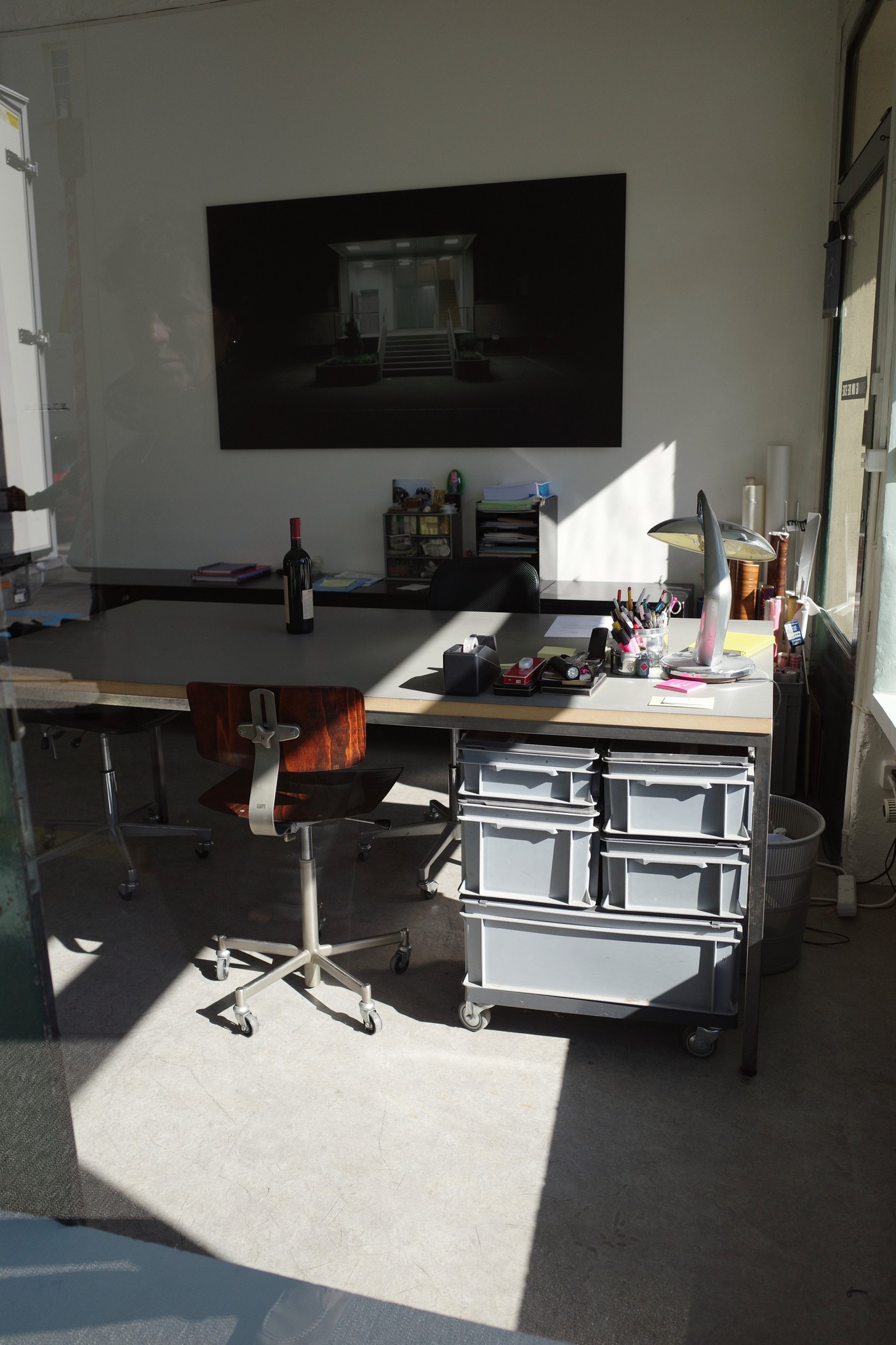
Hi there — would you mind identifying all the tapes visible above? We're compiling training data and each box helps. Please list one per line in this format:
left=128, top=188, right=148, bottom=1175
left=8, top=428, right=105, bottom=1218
left=463, top=637, right=479, bottom=652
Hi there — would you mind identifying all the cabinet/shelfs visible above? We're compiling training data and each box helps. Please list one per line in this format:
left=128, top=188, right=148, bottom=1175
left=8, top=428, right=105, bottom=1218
left=382, top=509, right=461, bottom=580
left=478, top=497, right=558, bottom=579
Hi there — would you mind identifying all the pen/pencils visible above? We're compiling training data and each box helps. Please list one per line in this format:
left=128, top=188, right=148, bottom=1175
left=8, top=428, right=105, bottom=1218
left=611, top=587, right=678, bottom=629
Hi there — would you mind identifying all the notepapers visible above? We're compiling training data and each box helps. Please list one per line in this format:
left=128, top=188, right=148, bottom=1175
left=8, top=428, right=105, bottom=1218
left=657, top=678, right=707, bottom=693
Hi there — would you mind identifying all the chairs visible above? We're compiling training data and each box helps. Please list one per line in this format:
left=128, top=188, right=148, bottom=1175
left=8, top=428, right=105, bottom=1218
left=428, top=558, right=542, bottom=613
left=186, top=680, right=411, bottom=1035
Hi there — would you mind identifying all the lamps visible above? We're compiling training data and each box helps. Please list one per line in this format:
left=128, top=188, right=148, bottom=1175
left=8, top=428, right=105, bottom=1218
left=651, top=491, right=778, bottom=680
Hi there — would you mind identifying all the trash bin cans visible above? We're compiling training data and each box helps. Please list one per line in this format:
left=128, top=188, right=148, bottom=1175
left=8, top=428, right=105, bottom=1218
left=762, top=795, right=826, bottom=971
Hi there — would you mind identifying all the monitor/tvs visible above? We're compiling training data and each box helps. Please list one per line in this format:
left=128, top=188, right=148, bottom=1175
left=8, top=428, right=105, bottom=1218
left=206, top=171, right=627, bottom=450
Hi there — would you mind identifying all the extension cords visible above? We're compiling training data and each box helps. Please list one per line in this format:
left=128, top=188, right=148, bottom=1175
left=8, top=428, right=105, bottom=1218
left=836, top=874, right=857, bottom=917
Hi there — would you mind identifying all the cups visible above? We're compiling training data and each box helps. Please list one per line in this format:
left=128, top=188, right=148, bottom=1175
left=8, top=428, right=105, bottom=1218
left=610, top=646, right=647, bottom=676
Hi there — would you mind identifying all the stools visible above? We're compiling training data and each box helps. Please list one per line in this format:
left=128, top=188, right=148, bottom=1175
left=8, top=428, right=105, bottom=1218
left=39, top=704, right=220, bottom=901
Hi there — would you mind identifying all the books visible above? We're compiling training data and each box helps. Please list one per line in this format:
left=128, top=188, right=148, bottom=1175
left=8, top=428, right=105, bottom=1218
left=192, top=562, right=271, bottom=582
left=477, top=499, right=538, bottom=558
left=656, top=678, right=706, bottom=693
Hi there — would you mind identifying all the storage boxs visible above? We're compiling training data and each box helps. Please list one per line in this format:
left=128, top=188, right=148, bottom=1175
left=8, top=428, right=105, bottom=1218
left=452, top=739, right=755, bottom=1019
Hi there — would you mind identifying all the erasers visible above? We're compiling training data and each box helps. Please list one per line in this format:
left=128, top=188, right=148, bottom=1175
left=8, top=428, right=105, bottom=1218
left=519, top=658, right=533, bottom=669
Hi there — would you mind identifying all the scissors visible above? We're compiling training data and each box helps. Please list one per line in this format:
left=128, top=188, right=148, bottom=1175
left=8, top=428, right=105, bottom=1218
left=660, top=591, right=682, bottom=614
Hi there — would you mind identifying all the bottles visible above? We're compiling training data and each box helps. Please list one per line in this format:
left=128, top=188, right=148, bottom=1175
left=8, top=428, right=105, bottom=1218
left=283, top=517, right=314, bottom=636
left=391, top=515, right=399, bottom=535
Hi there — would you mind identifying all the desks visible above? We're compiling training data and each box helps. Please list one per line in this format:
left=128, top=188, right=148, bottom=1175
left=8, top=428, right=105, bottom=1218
left=76, top=566, right=695, bottom=620
left=0, top=600, right=770, bottom=1087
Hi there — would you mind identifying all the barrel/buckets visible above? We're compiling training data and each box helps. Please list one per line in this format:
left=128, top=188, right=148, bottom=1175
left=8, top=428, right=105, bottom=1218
left=631, top=626, right=669, bottom=668
